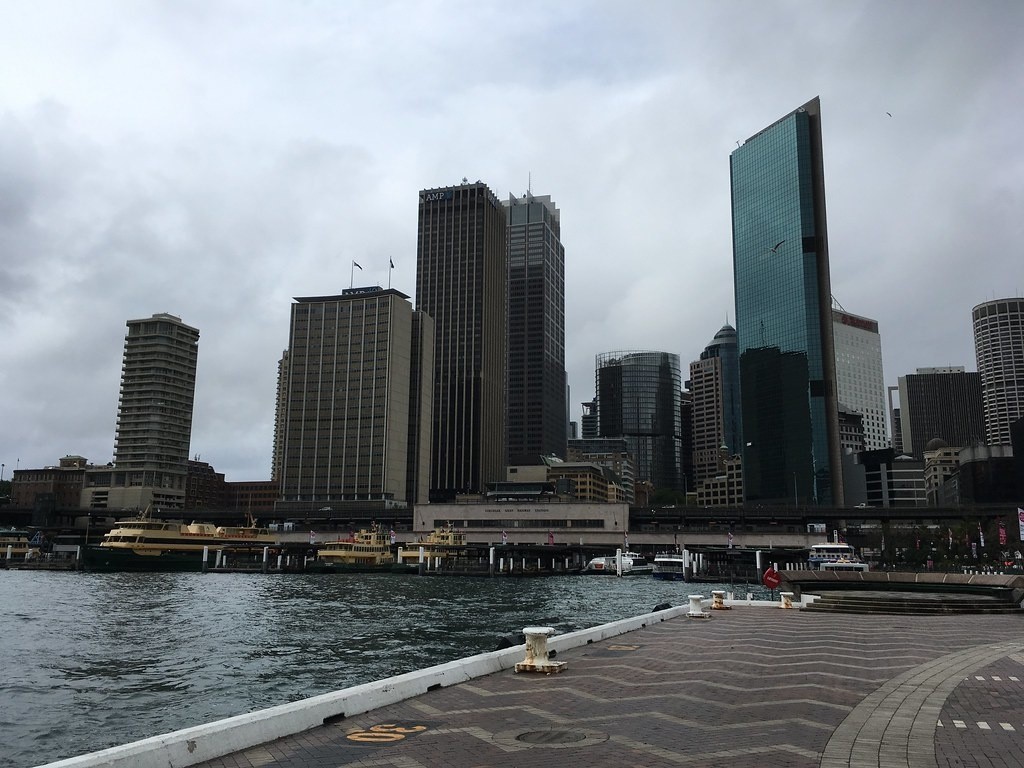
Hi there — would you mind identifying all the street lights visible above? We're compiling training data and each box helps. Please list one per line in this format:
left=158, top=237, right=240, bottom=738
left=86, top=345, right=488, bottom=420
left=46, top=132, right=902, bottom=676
left=1, top=464, right=5, bottom=493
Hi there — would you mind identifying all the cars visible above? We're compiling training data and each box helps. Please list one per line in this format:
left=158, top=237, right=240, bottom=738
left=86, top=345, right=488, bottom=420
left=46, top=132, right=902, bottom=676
left=319, top=506, right=333, bottom=512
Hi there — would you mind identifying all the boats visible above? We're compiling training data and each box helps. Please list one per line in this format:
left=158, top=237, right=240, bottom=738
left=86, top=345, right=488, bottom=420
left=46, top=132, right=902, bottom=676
left=807, top=544, right=856, bottom=563
left=400, top=522, right=469, bottom=574
left=81, top=505, right=286, bottom=573
left=653, top=554, right=684, bottom=581
left=587, top=551, right=650, bottom=575
left=0, top=530, right=73, bottom=570
left=819, top=556, right=870, bottom=573
left=318, top=520, right=395, bottom=572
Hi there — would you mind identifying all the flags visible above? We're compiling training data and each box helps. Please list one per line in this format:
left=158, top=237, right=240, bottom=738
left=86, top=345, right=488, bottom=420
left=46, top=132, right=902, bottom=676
left=310, top=530, right=316, bottom=544
left=390, top=529, right=397, bottom=544
left=728, top=534, right=734, bottom=550
left=354, top=261, right=362, bottom=271
left=625, top=532, right=629, bottom=548
left=502, top=530, right=508, bottom=545
left=548, top=531, right=554, bottom=546
left=389, top=259, right=395, bottom=268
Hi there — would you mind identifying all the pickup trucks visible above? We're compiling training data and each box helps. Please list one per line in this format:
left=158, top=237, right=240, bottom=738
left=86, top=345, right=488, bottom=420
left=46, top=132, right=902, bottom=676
left=854, top=503, right=876, bottom=509
